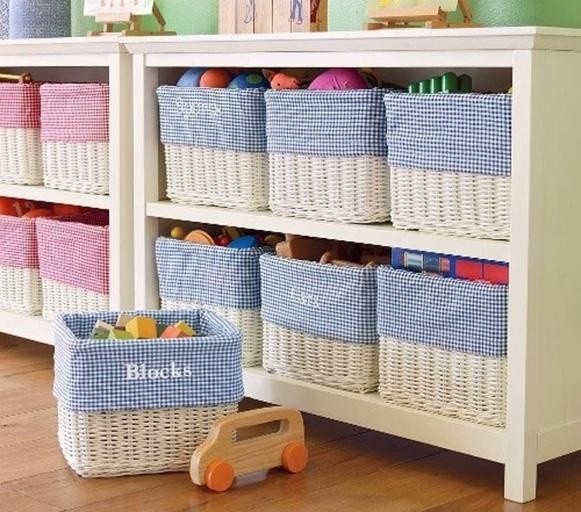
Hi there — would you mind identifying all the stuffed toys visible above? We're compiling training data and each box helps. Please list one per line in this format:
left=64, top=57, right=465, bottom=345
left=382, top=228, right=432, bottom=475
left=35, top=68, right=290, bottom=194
left=176, top=67, right=384, bottom=90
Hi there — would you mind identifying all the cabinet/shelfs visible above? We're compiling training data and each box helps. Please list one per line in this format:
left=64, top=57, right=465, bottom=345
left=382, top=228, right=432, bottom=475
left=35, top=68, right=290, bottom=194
left=2, top=35, right=132, bottom=347
left=118, top=36, right=580, bottom=504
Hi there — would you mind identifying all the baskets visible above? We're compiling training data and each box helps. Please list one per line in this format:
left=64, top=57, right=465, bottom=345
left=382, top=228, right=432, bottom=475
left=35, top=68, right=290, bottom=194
left=381, top=89, right=510, bottom=239
left=263, top=88, right=390, bottom=226
left=156, top=84, right=268, bottom=212
left=259, top=250, right=378, bottom=396
left=377, top=264, right=507, bottom=429
left=154, top=235, right=277, bottom=368
